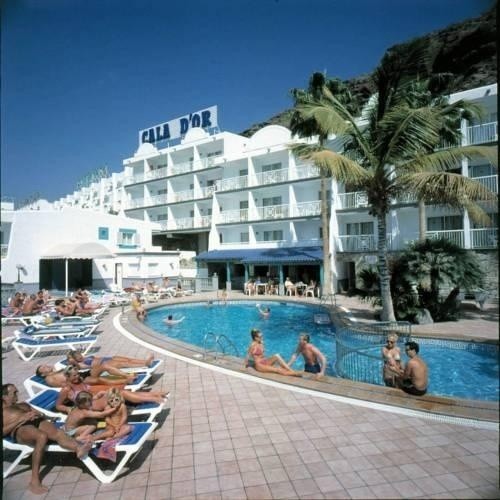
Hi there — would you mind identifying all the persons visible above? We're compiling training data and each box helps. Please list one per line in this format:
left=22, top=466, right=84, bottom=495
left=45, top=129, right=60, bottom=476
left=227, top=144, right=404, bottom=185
left=246, top=276, right=315, bottom=296
left=382, top=335, right=403, bottom=387
left=256, top=304, right=270, bottom=319
left=287, top=333, right=327, bottom=378
left=9, top=288, right=107, bottom=316
left=125, top=276, right=186, bottom=326
left=243, top=329, right=304, bottom=377
left=1, top=349, right=171, bottom=495
left=394, top=342, right=427, bottom=396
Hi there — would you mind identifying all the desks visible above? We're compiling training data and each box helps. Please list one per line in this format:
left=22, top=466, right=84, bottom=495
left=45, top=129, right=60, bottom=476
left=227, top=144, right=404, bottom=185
left=256, top=283, right=268, bottom=297
left=295, top=282, right=307, bottom=298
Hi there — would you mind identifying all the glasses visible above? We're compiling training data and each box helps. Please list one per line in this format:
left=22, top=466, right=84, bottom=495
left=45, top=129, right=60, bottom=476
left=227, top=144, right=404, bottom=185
left=386, top=340, right=394, bottom=343
left=109, top=398, right=119, bottom=402
left=68, top=364, right=75, bottom=370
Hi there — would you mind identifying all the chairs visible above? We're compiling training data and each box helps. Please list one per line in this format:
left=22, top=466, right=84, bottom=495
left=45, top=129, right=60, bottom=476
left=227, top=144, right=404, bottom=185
left=23, top=372, right=152, bottom=398
left=244, top=283, right=257, bottom=295
left=268, top=285, right=278, bottom=295
left=53, top=356, right=162, bottom=375
left=12, top=338, right=99, bottom=362
left=0, top=289, right=195, bottom=339
left=1, top=419, right=158, bottom=485
left=286, top=287, right=292, bottom=296
left=23, top=388, right=168, bottom=422
left=306, top=282, right=316, bottom=298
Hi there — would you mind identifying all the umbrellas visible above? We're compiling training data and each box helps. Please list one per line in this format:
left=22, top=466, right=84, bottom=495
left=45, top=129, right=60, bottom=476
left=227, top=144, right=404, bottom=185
left=35, top=242, right=117, bottom=297
